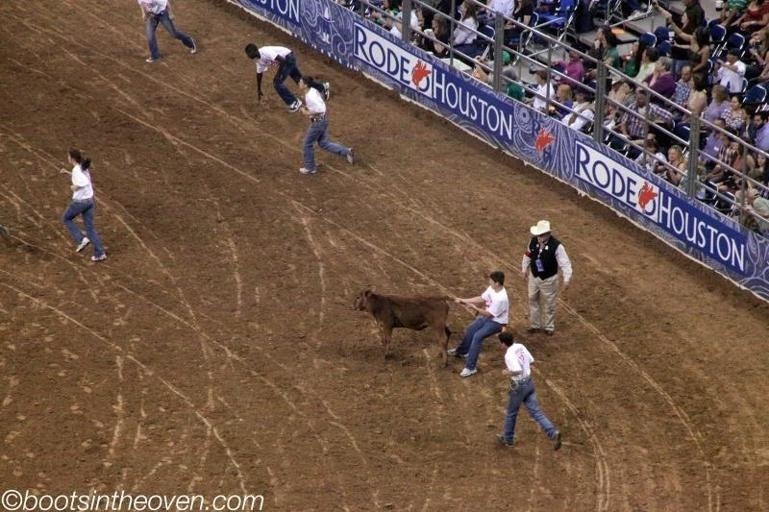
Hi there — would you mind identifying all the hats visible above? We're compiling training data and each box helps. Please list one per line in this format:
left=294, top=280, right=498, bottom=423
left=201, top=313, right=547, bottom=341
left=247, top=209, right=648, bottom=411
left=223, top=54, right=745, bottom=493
left=502, top=51, right=510, bottom=62
left=530, top=220, right=552, bottom=235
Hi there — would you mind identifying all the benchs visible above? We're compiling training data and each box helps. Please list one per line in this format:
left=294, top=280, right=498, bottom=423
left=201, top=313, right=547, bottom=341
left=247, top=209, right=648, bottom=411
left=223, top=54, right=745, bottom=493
left=341, top=0, right=578, bottom=101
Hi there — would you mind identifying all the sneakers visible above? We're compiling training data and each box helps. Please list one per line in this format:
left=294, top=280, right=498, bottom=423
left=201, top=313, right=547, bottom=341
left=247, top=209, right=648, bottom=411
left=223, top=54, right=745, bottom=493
left=447, top=348, right=469, bottom=357
left=91, top=254, right=107, bottom=261
left=460, top=367, right=477, bottom=377
left=527, top=327, right=540, bottom=331
left=288, top=97, right=302, bottom=112
left=323, top=81, right=329, bottom=100
left=190, top=39, right=197, bottom=53
left=76, top=237, right=90, bottom=253
left=347, top=148, right=354, bottom=164
left=145, top=56, right=161, bottom=63
left=496, top=434, right=514, bottom=447
left=300, top=168, right=316, bottom=174
left=545, top=330, right=552, bottom=335
left=552, top=430, right=561, bottom=450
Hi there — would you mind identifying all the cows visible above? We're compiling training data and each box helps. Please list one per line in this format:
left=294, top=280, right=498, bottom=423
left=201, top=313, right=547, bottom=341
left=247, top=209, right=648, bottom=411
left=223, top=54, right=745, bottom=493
left=348, top=286, right=467, bottom=369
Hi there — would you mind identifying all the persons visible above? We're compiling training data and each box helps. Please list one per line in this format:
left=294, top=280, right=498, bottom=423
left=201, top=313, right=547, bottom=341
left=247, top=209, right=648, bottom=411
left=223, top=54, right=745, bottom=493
left=447, top=271, right=510, bottom=378
left=335, top=0, right=768, bottom=241
left=498, top=331, right=561, bottom=450
left=521, top=220, right=572, bottom=336
left=60, top=148, right=108, bottom=261
left=299, top=75, right=354, bottom=174
left=137, top=0, right=197, bottom=64
left=245, top=42, right=330, bottom=112
